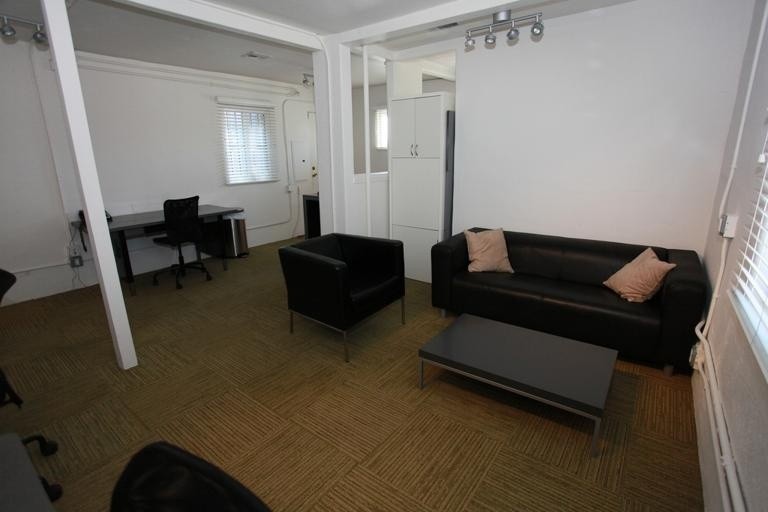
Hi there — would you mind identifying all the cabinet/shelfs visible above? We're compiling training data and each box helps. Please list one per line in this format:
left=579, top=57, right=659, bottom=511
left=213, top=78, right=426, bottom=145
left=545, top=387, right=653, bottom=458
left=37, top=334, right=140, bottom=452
left=300, top=192, right=322, bottom=242
left=388, top=92, right=447, bottom=288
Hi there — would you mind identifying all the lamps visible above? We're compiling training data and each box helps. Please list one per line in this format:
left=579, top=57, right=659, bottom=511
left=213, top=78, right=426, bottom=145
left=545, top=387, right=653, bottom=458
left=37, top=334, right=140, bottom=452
left=464, top=8, right=546, bottom=50
left=302, top=72, right=318, bottom=89
left=0, top=11, right=49, bottom=47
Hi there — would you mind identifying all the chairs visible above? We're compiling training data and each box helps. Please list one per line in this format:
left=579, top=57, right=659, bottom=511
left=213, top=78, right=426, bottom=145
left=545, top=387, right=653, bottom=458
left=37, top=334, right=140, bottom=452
left=107, top=437, right=275, bottom=511
left=150, top=194, right=215, bottom=292
left=0, top=267, right=27, bottom=410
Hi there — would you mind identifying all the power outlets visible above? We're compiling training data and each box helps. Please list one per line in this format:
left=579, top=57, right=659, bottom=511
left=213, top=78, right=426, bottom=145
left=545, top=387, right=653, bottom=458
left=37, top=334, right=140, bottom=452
left=70, top=256, right=85, bottom=269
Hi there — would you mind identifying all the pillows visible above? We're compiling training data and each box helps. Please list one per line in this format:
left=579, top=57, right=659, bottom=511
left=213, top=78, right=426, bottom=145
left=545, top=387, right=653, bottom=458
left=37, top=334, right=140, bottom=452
left=600, top=244, right=679, bottom=306
left=463, top=226, right=515, bottom=277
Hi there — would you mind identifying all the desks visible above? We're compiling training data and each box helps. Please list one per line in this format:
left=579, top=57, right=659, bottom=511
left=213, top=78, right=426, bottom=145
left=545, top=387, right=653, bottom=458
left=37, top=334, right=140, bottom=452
left=70, top=202, right=248, bottom=299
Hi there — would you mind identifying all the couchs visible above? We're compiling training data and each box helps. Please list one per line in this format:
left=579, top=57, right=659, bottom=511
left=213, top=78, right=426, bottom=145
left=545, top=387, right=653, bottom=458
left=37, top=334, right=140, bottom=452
left=276, top=230, right=406, bottom=362
left=428, top=226, right=712, bottom=380
